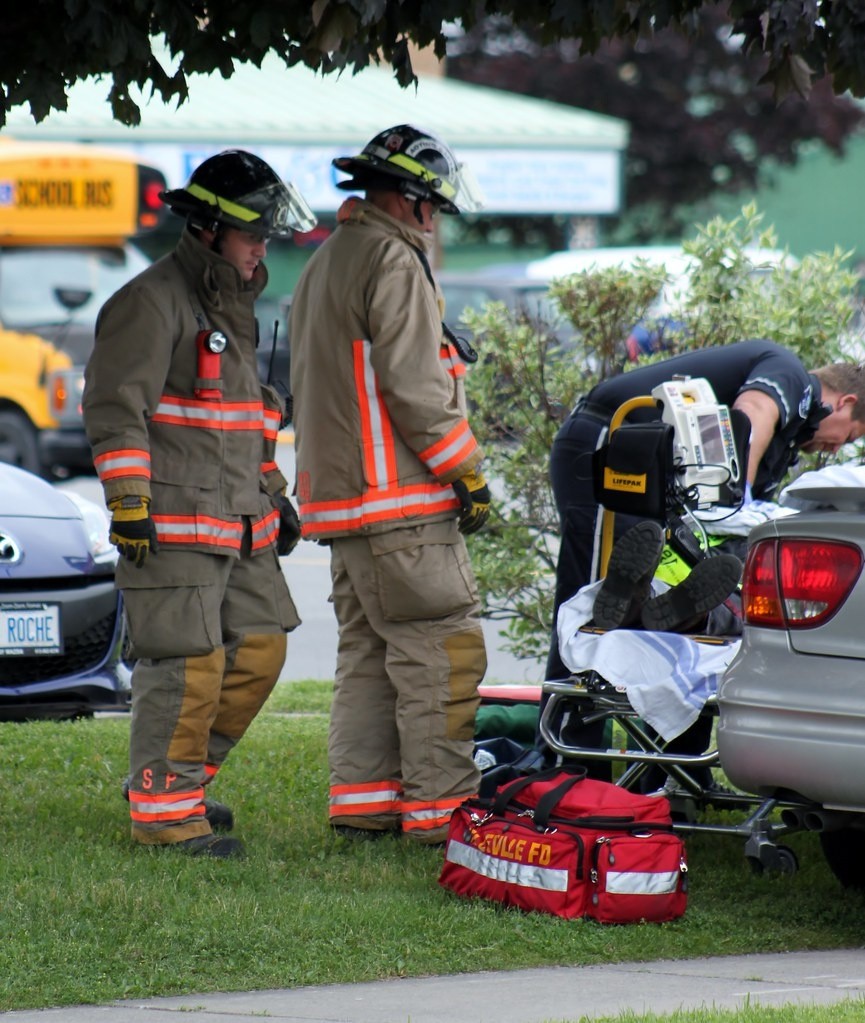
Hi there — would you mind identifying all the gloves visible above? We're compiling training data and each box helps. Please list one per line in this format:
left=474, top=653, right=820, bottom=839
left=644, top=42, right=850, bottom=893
left=273, top=486, right=301, bottom=556
left=107, top=495, right=160, bottom=569
left=452, top=465, right=490, bottom=535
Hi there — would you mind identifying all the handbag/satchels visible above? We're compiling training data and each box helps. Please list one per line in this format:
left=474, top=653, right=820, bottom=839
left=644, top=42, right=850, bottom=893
left=438, top=764, right=690, bottom=925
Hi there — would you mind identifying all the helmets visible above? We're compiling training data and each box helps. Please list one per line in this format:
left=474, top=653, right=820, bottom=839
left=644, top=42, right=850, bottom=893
left=332, top=125, right=462, bottom=215
left=159, top=150, right=292, bottom=238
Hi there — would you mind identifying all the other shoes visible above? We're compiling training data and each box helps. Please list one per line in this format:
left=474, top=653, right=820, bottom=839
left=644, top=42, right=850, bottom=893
left=592, top=520, right=658, bottom=630
left=121, top=775, right=234, bottom=833
left=335, top=825, right=400, bottom=845
left=704, top=783, right=751, bottom=813
left=174, top=834, right=246, bottom=861
left=641, top=555, right=743, bottom=634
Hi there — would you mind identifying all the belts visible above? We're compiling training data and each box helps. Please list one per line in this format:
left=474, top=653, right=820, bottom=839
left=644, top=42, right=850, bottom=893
left=572, top=402, right=630, bottom=428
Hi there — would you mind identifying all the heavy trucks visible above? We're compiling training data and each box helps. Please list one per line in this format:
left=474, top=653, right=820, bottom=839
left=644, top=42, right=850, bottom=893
left=1, top=154, right=174, bottom=484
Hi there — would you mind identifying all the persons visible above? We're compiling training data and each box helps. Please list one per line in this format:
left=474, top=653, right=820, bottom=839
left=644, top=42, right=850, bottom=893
left=536, top=340, right=865, bottom=812
left=290, top=124, right=492, bottom=843
left=594, top=500, right=796, bottom=634
left=82, top=149, right=311, bottom=857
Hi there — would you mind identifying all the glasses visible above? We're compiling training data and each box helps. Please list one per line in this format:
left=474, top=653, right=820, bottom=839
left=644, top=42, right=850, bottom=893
left=243, top=233, right=270, bottom=245
left=430, top=208, right=441, bottom=219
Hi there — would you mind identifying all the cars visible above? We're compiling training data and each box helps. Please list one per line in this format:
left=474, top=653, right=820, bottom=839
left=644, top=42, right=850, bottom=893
left=279, top=244, right=865, bottom=435
left=715, top=457, right=864, bottom=893
left=1, top=462, right=139, bottom=726
